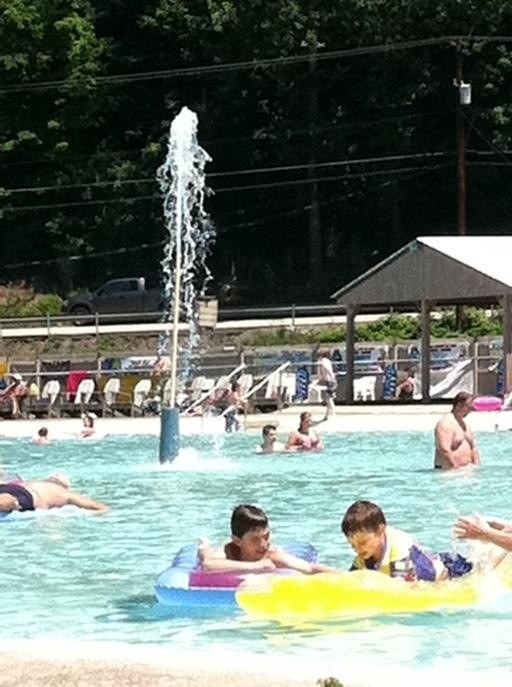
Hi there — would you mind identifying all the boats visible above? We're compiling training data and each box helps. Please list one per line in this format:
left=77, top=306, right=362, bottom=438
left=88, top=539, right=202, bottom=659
left=154, top=541, right=316, bottom=608
left=235, top=549, right=512, bottom=622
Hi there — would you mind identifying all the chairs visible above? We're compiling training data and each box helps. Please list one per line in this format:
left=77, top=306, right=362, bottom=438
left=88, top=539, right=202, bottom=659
left=25, top=370, right=379, bottom=417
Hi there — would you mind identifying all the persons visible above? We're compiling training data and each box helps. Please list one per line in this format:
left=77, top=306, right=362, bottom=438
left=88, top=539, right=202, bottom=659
left=433, top=392, right=482, bottom=470
left=394, top=366, right=417, bottom=400
left=317, top=500, right=498, bottom=584
left=2, top=364, right=26, bottom=416
left=31, top=426, right=50, bottom=445
left=194, top=502, right=336, bottom=576
left=315, top=350, right=339, bottom=420
left=1, top=471, right=105, bottom=515
left=287, top=412, right=322, bottom=452
left=78, top=415, right=97, bottom=439
left=149, top=349, right=170, bottom=376
left=224, top=382, right=248, bottom=434
left=253, top=424, right=285, bottom=451
left=453, top=509, right=512, bottom=556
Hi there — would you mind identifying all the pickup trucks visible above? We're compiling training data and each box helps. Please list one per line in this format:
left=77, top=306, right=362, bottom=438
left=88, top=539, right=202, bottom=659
left=60, top=275, right=219, bottom=325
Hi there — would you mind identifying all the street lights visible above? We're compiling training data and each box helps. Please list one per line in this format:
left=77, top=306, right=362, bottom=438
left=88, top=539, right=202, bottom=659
left=451, top=78, right=474, bottom=233
